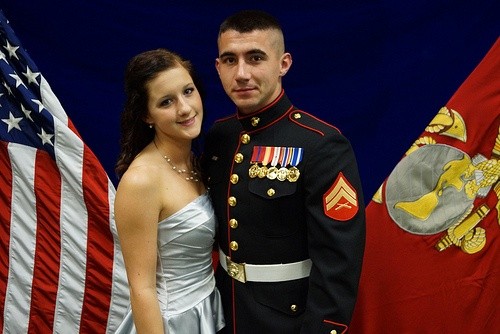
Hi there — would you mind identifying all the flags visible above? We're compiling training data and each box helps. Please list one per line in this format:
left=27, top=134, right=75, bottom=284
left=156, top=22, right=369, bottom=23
left=0, top=11, right=132, bottom=334
left=347, top=35, right=500, bottom=334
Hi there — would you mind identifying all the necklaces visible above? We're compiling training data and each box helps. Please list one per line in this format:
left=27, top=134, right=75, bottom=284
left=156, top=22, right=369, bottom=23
left=153, top=139, right=202, bottom=185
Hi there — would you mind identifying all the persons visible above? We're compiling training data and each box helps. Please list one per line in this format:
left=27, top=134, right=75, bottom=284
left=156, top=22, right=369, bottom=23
left=189, top=11, right=369, bottom=333
left=113, top=50, right=228, bottom=334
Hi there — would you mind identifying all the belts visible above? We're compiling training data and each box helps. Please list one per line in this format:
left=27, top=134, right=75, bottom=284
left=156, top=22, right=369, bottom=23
left=217, top=249, right=318, bottom=285
